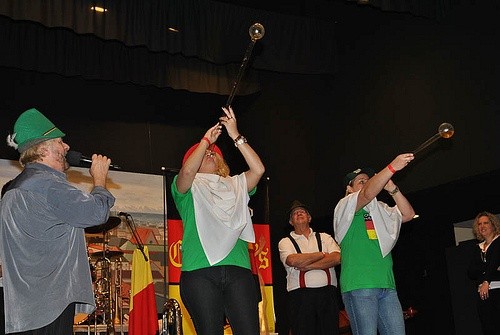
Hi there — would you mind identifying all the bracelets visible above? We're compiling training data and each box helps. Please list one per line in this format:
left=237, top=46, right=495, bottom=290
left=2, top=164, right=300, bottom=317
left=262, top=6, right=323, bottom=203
left=234, top=136, right=248, bottom=147
left=321, top=251, right=326, bottom=256
left=388, top=185, right=399, bottom=196
left=233, top=134, right=241, bottom=143
left=387, top=164, right=397, bottom=174
left=201, top=136, right=211, bottom=148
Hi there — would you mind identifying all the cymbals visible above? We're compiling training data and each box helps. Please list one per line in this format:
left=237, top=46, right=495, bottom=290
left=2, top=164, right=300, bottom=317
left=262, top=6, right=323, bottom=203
left=92, top=250, right=125, bottom=258
left=85, top=216, right=122, bottom=234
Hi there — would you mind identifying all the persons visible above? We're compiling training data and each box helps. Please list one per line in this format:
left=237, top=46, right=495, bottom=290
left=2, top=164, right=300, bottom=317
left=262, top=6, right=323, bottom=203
left=0, top=108, right=117, bottom=335
left=277, top=200, right=341, bottom=335
left=333, top=153, right=416, bottom=335
left=472, top=210, right=500, bottom=335
left=170, top=105, right=266, bottom=335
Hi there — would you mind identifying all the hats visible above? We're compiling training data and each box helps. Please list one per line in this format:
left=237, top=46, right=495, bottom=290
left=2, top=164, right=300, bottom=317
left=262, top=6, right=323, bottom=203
left=182, top=142, right=223, bottom=167
left=8, top=108, right=66, bottom=153
left=344, top=168, right=377, bottom=185
left=287, top=200, right=311, bottom=218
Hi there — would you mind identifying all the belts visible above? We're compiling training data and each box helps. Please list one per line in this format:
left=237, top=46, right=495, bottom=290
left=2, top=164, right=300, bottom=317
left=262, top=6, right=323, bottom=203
left=287, top=285, right=336, bottom=295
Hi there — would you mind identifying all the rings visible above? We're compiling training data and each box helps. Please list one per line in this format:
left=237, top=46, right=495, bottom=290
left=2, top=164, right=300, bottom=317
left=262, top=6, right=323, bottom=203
left=482, top=296, right=484, bottom=298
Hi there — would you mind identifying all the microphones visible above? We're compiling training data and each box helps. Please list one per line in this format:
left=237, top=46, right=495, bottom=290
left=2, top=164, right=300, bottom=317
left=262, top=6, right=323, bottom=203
left=116, top=210, right=132, bottom=217
left=66, top=150, right=122, bottom=170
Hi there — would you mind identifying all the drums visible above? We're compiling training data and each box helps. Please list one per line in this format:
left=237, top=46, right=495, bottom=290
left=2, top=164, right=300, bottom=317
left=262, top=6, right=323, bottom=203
left=72, top=264, right=110, bottom=325
